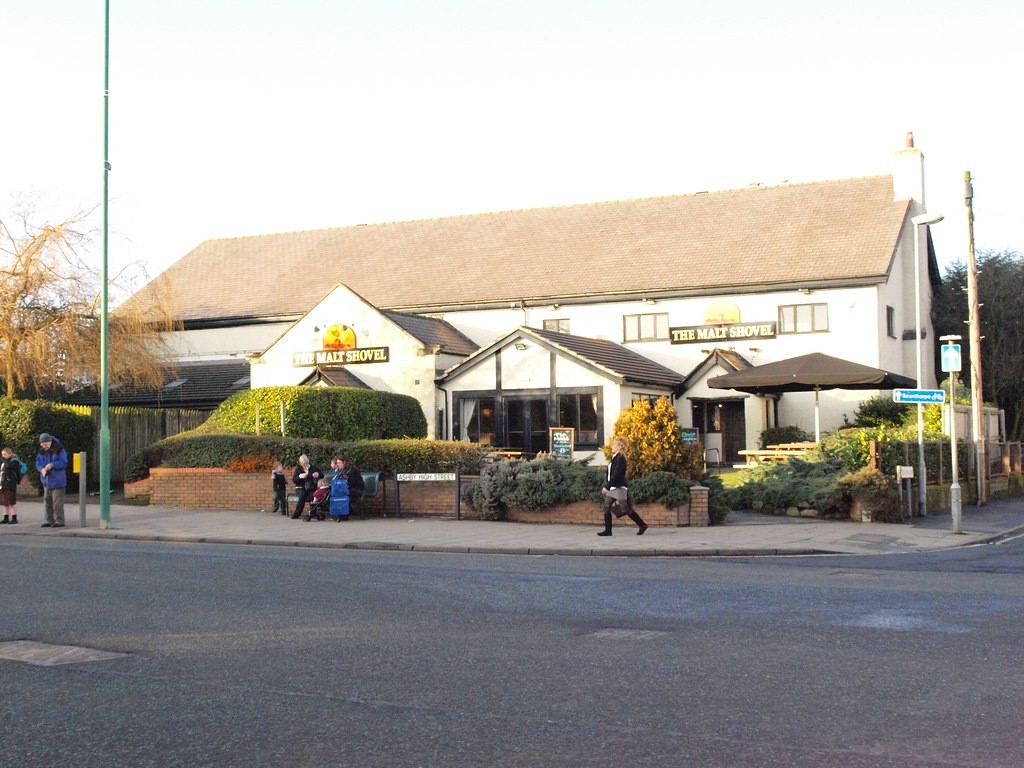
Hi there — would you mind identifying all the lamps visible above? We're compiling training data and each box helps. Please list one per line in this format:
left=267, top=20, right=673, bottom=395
left=342, top=323, right=354, bottom=332
left=313, top=325, right=326, bottom=333
left=547, top=305, right=556, bottom=311
left=515, top=343, right=526, bottom=350
left=800, top=289, right=810, bottom=294
left=229, top=352, right=237, bottom=356
left=645, top=299, right=656, bottom=305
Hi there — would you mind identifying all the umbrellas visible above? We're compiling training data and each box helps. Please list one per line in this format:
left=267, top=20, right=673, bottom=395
left=706, top=353, right=917, bottom=444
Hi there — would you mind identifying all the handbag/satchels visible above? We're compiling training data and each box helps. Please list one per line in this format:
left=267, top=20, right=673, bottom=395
left=611, top=502, right=627, bottom=518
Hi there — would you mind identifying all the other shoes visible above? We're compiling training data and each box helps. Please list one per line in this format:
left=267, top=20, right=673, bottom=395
left=51, top=524, right=65, bottom=528
left=636, top=526, right=648, bottom=535
left=597, top=530, right=612, bottom=536
left=0, top=520, right=9, bottom=524
left=9, top=520, right=18, bottom=524
left=41, top=523, right=51, bottom=527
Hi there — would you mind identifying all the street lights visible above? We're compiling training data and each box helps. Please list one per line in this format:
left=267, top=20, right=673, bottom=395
left=911, top=213, right=946, bottom=516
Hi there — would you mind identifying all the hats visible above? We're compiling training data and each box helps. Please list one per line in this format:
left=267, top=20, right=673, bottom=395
left=39, top=433, right=51, bottom=443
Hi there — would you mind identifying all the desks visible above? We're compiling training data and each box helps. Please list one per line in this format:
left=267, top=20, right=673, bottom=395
left=735, top=449, right=807, bottom=465
left=765, top=443, right=816, bottom=451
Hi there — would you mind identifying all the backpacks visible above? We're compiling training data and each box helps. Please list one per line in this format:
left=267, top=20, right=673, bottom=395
left=10, top=458, right=27, bottom=485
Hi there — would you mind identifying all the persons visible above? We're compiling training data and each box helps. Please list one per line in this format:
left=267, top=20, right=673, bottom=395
left=330, top=456, right=341, bottom=474
left=303, top=478, right=327, bottom=522
left=291, top=454, right=324, bottom=519
left=336, top=455, right=365, bottom=522
left=597, top=436, right=648, bottom=536
left=0, top=447, right=18, bottom=524
left=36, top=433, right=68, bottom=528
left=271, top=461, right=292, bottom=515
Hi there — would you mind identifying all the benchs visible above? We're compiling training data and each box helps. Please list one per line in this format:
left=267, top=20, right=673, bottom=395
left=767, top=455, right=804, bottom=460
left=733, top=464, right=763, bottom=469
left=285, top=470, right=387, bottom=520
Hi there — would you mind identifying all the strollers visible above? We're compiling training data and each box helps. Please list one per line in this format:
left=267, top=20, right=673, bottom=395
left=301, top=464, right=354, bottom=522
